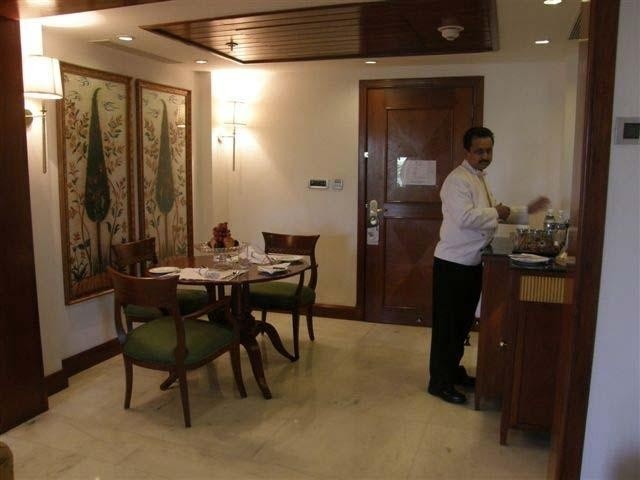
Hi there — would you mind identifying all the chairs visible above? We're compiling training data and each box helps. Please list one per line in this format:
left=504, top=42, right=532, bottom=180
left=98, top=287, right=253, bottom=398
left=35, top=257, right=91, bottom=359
left=243, top=231, right=320, bottom=359
left=109, top=236, right=210, bottom=380
left=105, top=265, right=248, bottom=427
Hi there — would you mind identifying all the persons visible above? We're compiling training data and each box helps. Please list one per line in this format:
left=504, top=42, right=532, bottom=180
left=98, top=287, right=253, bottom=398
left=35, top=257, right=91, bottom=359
left=423, top=124, right=554, bottom=406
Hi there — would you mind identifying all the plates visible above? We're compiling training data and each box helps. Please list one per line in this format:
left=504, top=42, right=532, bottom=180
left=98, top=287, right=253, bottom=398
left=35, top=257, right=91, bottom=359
left=272, top=255, right=305, bottom=262
left=508, top=253, right=550, bottom=264
left=147, top=266, right=180, bottom=275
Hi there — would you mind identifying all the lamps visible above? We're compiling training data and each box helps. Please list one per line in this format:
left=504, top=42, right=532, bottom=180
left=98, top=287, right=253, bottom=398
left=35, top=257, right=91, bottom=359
left=23, top=53, right=63, bottom=175
left=217, top=99, right=248, bottom=172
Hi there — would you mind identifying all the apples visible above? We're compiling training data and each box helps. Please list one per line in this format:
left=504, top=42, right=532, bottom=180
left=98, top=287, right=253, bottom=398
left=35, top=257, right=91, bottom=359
left=208, top=237, right=239, bottom=247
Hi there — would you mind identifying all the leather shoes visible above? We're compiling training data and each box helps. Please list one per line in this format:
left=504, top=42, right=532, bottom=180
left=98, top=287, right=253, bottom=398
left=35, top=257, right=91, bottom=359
left=459, top=375, right=476, bottom=386
left=436, top=386, right=466, bottom=403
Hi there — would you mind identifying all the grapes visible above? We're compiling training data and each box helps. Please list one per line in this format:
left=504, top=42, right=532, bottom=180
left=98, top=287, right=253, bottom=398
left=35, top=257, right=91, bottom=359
left=213, top=222, right=231, bottom=242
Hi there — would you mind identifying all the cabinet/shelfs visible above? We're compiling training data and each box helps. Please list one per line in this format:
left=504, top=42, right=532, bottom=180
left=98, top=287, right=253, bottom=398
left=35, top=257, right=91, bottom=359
left=474, top=244, right=578, bottom=446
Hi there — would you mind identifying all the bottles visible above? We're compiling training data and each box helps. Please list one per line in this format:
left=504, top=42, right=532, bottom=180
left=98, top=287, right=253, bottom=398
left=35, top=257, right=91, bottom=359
left=543, top=209, right=567, bottom=233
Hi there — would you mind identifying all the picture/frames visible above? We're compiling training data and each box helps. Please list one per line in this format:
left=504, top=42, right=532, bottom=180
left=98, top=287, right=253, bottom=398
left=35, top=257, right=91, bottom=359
left=57, top=59, right=138, bottom=307
left=134, top=76, right=194, bottom=264
